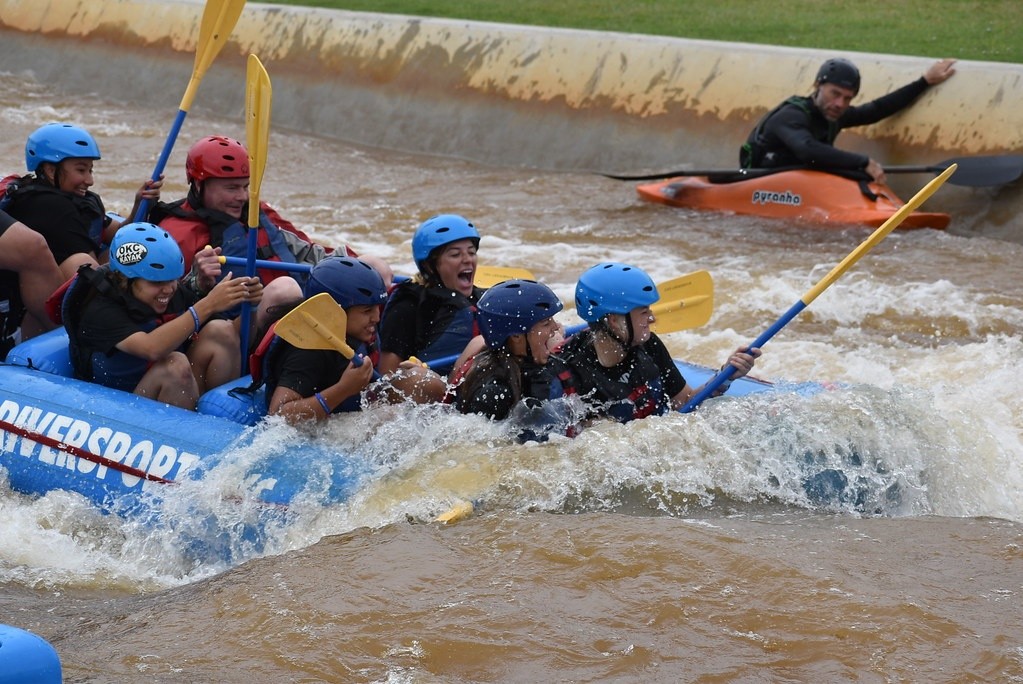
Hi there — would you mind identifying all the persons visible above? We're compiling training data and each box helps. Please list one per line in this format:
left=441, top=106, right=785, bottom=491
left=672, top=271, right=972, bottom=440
left=64, top=222, right=265, bottom=412
left=1, top=121, right=164, bottom=348
left=458, top=278, right=568, bottom=441
left=737, top=57, right=960, bottom=185
left=264, top=213, right=495, bottom=425
left=151, top=135, right=396, bottom=356
left=548, top=261, right=763, bottom=423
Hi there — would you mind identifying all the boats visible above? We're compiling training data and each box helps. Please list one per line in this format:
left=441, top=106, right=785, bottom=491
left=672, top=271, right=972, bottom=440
left=0, top=327, right=937, bottom=573
left=636, top=168, right=954, bottom=232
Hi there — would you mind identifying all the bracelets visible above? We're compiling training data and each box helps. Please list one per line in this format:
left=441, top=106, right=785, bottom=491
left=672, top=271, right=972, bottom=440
left=314, top=392, right=332, bottom=415
left=188, top=307, right=200, bottom=334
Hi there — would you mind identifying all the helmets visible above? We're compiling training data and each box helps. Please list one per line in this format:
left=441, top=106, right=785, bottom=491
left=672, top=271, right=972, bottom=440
left=476, top=279, right=563, bottom=351
left=575, top=262, right=660, bottom=322
left=815, top=57, right=860, bottom=98
left=109, top=222, right=185, bottom=281
left=185, top=135, right=250, bottom=185
left=304, top=255, right=388, bottom=308
left=412, top=214, right=480, bottom=280
left=26, top=123, right=102, bottom=171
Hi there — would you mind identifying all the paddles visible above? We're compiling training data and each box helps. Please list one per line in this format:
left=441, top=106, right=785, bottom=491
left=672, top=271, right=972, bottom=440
left=595, top=152, right=1023, bottom=190
left=274, top=291, right=383, bottom=382
left=133, top=0, right=248, bottom=222
left=408, top=265, right=714, bottom=369
left=239, top=49, right=276, bottom=377
left=200, top=243, right=538, bottom=288
left=678, top=162, right=960, bottom=414
